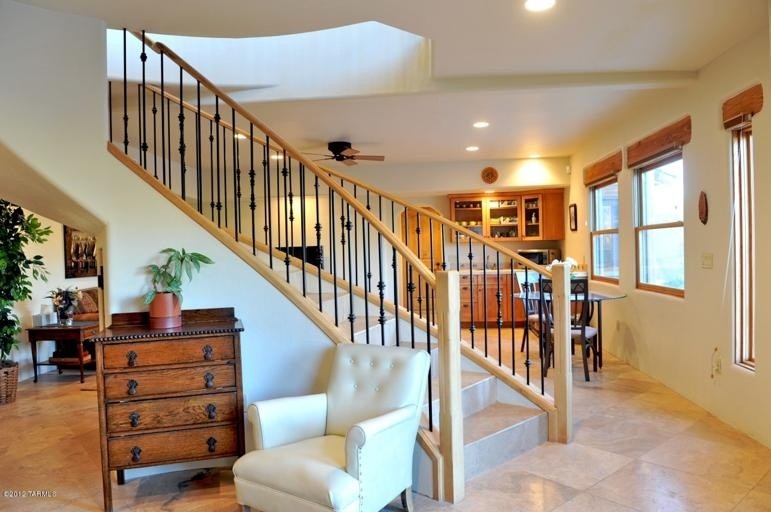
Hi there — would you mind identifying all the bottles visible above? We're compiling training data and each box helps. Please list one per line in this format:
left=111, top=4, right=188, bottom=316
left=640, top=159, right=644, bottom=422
left=530, top=212, right=537, bottom=224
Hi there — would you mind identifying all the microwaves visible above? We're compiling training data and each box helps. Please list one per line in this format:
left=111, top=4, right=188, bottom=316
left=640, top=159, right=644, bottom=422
left=515, top=249, right=549, bottom=271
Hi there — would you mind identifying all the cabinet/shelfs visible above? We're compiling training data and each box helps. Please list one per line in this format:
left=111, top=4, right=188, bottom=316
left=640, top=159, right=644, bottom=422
left=458, top=274, right=539, bottom=329
left=88, top=306, right=249, bottom=511
left=447, top=188, right=565, bottom=244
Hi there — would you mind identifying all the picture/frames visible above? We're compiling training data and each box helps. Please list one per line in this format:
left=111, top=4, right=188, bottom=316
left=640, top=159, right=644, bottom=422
left=62, top=224, right=98, bottom=280
left=569, top=203, right=577, bottom=231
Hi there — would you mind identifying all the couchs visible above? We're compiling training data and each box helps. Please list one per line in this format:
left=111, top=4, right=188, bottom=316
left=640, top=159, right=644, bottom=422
left=68, top=285, right=100, bottom=323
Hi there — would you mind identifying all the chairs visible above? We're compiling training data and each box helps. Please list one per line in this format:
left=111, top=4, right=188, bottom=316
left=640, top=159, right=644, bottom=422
left=229, top=340, right=432, bottom=512
left=515, top=268, right=599, bottom=381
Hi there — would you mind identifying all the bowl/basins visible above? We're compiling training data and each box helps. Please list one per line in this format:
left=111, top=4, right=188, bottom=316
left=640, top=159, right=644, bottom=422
left=454, top=221, right=482, bottom=227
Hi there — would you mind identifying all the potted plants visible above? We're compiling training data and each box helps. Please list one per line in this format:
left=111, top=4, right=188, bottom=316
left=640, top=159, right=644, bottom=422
left=137, top=247, right=214, bottom=330
left=0, top=199, right=54, bottom=405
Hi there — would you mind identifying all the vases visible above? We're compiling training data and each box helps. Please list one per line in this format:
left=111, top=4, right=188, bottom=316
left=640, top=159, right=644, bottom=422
left=56, top=310, right=74, bottom=326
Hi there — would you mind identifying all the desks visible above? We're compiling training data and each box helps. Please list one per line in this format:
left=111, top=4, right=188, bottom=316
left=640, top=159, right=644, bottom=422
left=511, top=290, right=627, bottom=372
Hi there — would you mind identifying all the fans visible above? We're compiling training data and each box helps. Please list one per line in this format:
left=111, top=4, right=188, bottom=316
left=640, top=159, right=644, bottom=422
left=298, top=140, right=386, bottom=167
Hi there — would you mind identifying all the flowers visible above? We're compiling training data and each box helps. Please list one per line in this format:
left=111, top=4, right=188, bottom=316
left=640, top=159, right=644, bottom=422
left=43, top=286, right=84, bottom=316
